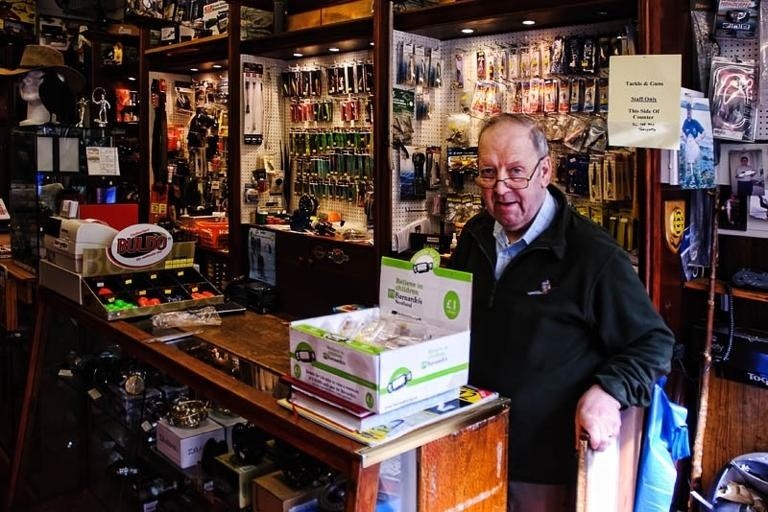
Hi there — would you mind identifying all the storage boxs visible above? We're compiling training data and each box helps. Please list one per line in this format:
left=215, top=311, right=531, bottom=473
left=288, top=247, right=472, bottom=416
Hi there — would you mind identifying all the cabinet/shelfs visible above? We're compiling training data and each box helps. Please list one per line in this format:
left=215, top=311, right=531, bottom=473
left=79, top=30, right=140, bottom=203
left=8, top=124, right=115, bottom=277
left=242, top=224, right=373, bottom=320
left=684, top=228, right=768, bottom=512
left=0, top=287, right=509, bottom=511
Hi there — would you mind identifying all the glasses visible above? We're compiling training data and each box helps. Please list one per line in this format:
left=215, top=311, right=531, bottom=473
left=474, top=157, right=544, bottom=190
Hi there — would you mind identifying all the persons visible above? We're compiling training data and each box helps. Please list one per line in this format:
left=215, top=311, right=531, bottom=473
left=736, top=157, right=755, bottom=196
left=682, top=103, right=706, bottom=144
left=446, top=113, right=675, bottom=512
